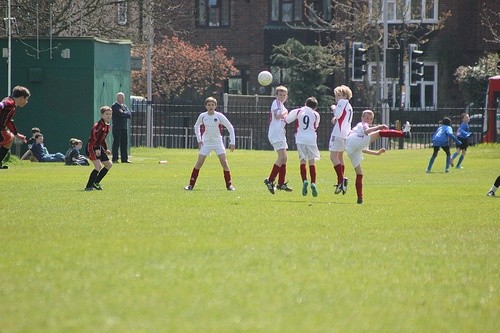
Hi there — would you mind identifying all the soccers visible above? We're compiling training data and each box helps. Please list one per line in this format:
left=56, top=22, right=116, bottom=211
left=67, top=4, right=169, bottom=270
left=258, top=71, right=273, bottom=86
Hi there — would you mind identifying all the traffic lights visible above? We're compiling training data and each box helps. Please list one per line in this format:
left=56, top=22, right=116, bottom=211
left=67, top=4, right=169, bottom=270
left=353, top=41, right=367, bottom=82
left=408, top=44, right=424, bottom=86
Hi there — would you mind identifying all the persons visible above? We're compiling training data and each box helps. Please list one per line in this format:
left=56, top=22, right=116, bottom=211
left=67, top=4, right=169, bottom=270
left=329, top=85, right=411, bottom=204
left=486, top=176, right=500, bottom=196
left=0, top=85, right=30, bottom=169
left=184, top=97, right=236, bottom=190
left=111, top=92, right=133, bottom=163
left=425, top=118, right=462, bottom=173
left=24, top=128, right=90, bottom=166
left=85, top=105, right=113, bottom=190
left=450, top=113, right=470, bottom=169
left=280, top=96, right=320, bottom=197
left=264, top=85, right=292, bottom=194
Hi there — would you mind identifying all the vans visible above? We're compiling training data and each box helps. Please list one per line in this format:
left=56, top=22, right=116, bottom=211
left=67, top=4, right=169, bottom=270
left=467, top=113, right=500, bottom=135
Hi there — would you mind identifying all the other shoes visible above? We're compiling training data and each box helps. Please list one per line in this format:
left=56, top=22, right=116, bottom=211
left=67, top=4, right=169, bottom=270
left=93, top=182, right=102, bottom=190
left=85, top=187, right=94, bottom=191
left=332, top=184, right=341, bottom=195
left=311, top=183, right=318, bottom=197
left=403, top=120, right=412, bottom=138
left=113, top=160, right=119, bottom=162
left=184, top=185, right=193, bottom=190
left=227, top=186, right=234, bottom=190
left=263, top=178, right=275, bottom=194
left=121, top=161, right=131, bottom=162
left=276, top=180, right=292, bottom=192
left=339, top=178, right=349, bottom=195
left=450, top=157, right=454, bottom=167
left=302, top=180, right=308, bottom=196
left=488, top=191, right=495, bottom=197
